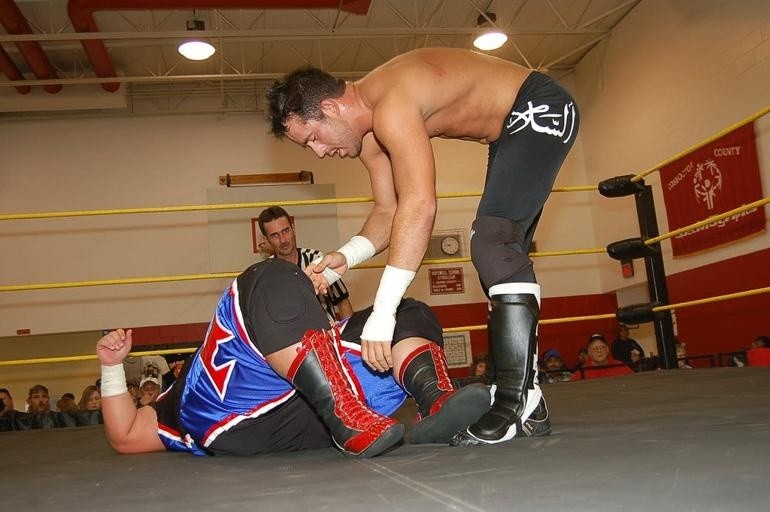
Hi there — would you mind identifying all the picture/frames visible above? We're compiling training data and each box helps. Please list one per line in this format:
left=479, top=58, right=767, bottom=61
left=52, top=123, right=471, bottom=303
left=251, top=216, right=296, bottom=253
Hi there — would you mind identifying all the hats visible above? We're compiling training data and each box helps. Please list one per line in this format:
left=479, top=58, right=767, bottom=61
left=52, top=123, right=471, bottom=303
left=589, top=334, right=604, bottom=346
left=139, top=377, right=160, bottom=389
left=544, top=350, right=559, bottom=363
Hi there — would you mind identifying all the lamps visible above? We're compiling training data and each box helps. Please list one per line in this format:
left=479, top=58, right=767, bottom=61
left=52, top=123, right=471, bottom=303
left=177, top=9, right=216, bottom=60
left=473, top=0, right=508, bottom=51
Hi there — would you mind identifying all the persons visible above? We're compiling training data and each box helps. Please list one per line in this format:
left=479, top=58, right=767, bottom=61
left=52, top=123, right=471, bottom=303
left=734, top=336, right=770, bottom=366
left=0, top=350, right=193, bottom=433
left=537, top=324, right=646, bottom=383
left=257, top=205, right=355, bottom=322
left=263, top=45, right=582, bottom=447
left=95, top=258, right=493, bottom=461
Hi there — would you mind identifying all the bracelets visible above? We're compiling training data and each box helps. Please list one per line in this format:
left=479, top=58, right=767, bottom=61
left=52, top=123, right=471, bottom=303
left=335, top=236, right=376, bottom=269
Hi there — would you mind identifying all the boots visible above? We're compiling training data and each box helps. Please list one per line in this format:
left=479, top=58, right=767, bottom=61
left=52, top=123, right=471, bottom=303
left=451, top=283, right=551, bottom=445
left=287, top=325, right=406, bottom=456
left=399, top=342, right=492, bottom=444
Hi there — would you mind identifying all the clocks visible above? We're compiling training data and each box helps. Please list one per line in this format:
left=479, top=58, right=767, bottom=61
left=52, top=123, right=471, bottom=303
left=440, top=235, right=460, bottom=255
